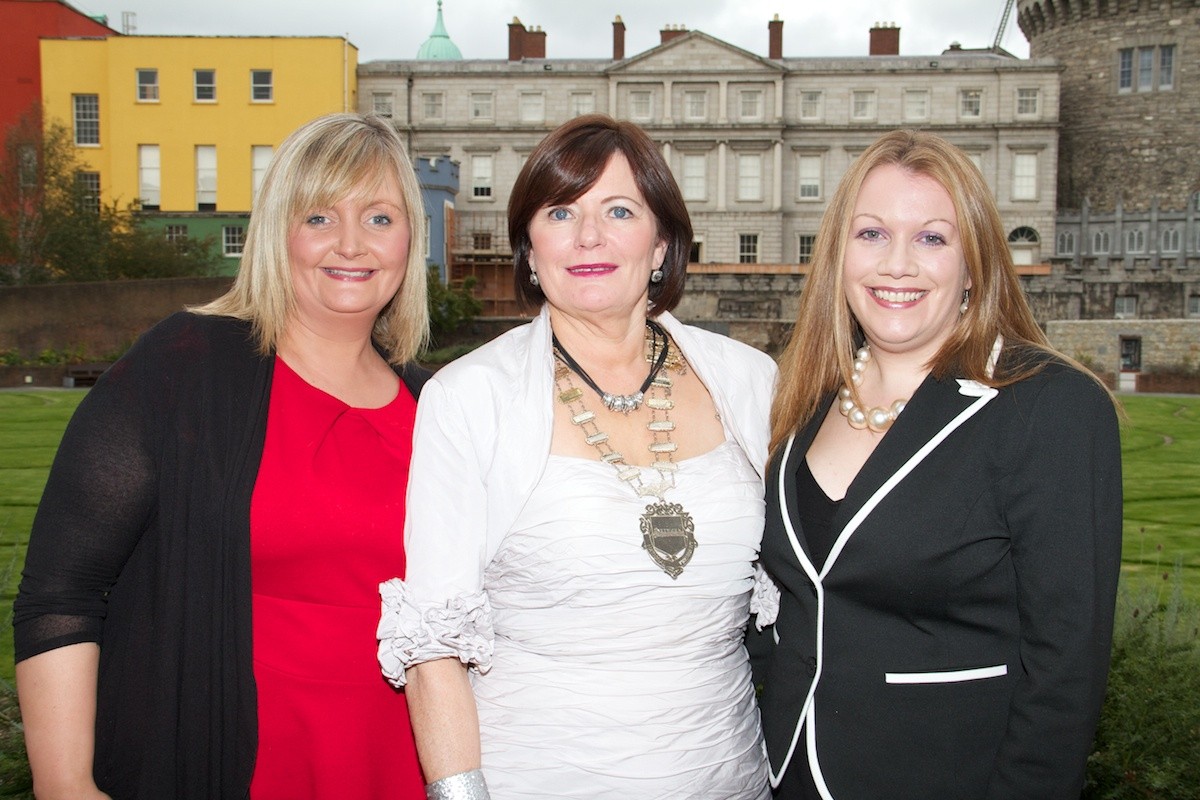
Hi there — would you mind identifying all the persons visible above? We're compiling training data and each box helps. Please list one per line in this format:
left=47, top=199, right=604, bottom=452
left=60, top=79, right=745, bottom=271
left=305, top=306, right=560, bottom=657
left=13, top=111, right=441, bottom=800
left=745, top=129, right=1123, bottom=800
left=374, top=113, right=783, bottom=800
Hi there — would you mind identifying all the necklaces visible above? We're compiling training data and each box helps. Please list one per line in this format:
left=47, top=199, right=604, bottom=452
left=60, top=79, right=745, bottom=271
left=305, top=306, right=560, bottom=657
left=551, top=322, right=668, bottom=414
left=838, top=342, right=907, bottom=432
left=555, top=332, right=698, bottom=579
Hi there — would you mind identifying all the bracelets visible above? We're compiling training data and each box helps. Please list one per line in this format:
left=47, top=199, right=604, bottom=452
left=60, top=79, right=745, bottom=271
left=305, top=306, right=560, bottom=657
left=425, top=770, right=491, bottom=800
